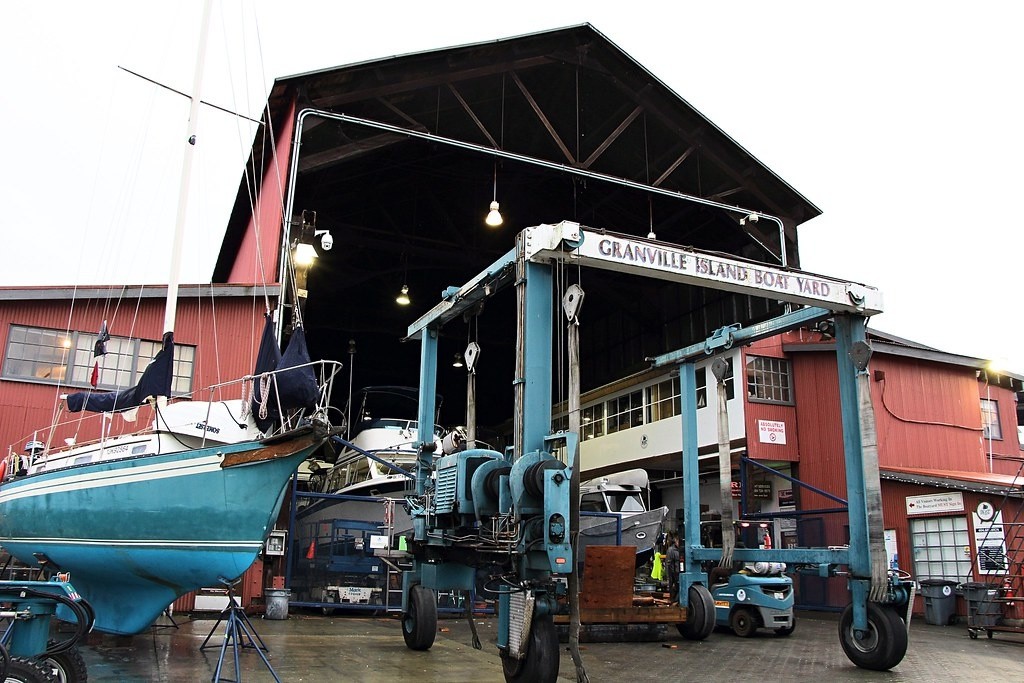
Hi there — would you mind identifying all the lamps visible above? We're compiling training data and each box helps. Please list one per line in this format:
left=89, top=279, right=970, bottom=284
left=648, top=197, right=656, bottom=240
left=396, top=284, right=410, bottom=305
left=484, top=156, right=504, bottom=227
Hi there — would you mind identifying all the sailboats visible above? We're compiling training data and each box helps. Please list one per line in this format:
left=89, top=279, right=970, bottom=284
left=2, top=66, right=344, bottom=641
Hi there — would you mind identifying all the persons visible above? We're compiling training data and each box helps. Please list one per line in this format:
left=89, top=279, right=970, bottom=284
left=666, top=532, right=683, bottom=605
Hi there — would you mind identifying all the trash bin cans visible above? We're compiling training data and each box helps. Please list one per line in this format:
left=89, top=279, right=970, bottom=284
left=918, top=578, right=962, bottom=626
left=264, top=586, right=292, bottom=620
left=960, top=582, right=1005, bottom=626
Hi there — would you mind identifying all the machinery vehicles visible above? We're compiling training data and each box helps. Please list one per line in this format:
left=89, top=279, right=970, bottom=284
left=398, top=219, right=912, bottom=683
left=690, top=516, right=797, bottom=638
left=294, top=517, right=390, bottom=618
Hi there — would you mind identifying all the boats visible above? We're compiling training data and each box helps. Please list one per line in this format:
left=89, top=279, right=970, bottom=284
left=290, top=383, right=448, bottom=561
left=567, top=469, right=669, bottom=569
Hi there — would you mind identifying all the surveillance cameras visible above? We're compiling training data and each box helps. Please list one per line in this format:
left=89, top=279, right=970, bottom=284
left=314, top=228, right=333, bottom=251
